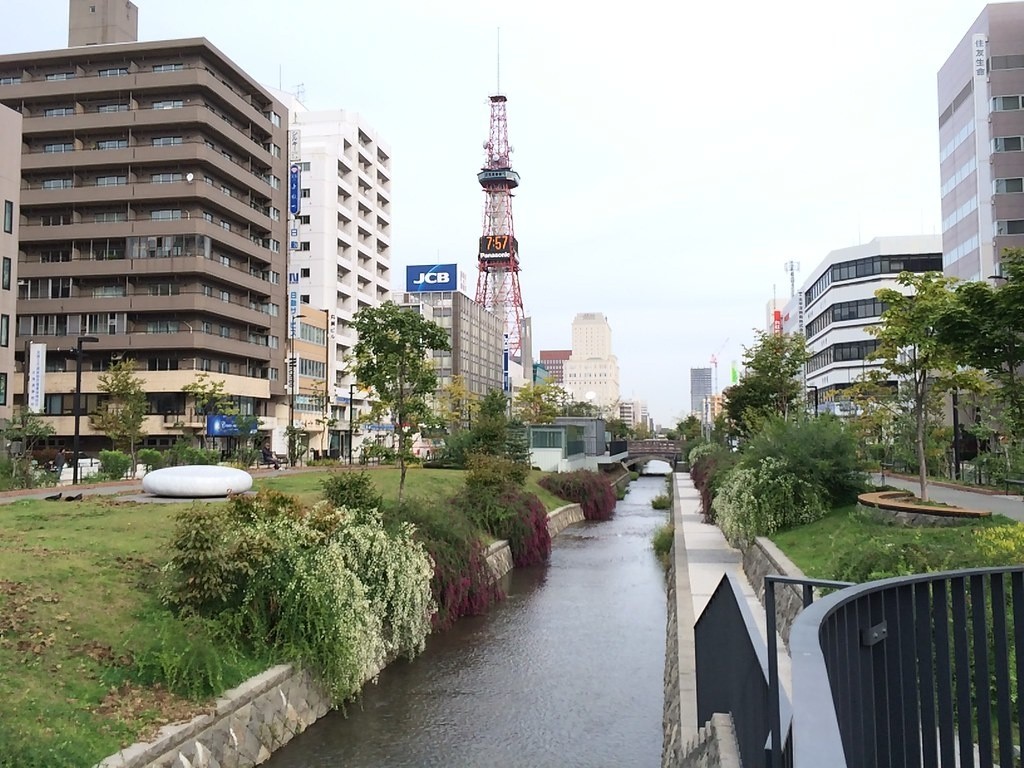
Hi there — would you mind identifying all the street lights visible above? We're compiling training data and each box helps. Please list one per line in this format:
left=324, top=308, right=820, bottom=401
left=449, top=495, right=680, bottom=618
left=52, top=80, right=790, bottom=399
left=348, top=383, right=363, bottom=464
left=805, top=383, right=819, bottom=419
left=291, top=314, right=307, bottom=426
left=72, top=336, right=100, bottom=484
left=468, top=403, right=476, bottom=431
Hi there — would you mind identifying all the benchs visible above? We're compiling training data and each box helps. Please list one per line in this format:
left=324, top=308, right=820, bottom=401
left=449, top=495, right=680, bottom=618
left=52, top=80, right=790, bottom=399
left=1005, top=471, right=1024, bottom=502
left=257, top=451, right=288, bottom=469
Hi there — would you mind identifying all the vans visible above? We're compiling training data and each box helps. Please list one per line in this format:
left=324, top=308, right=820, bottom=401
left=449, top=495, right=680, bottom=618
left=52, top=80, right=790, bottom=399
left=394, top=423, right=447, bottom=461
